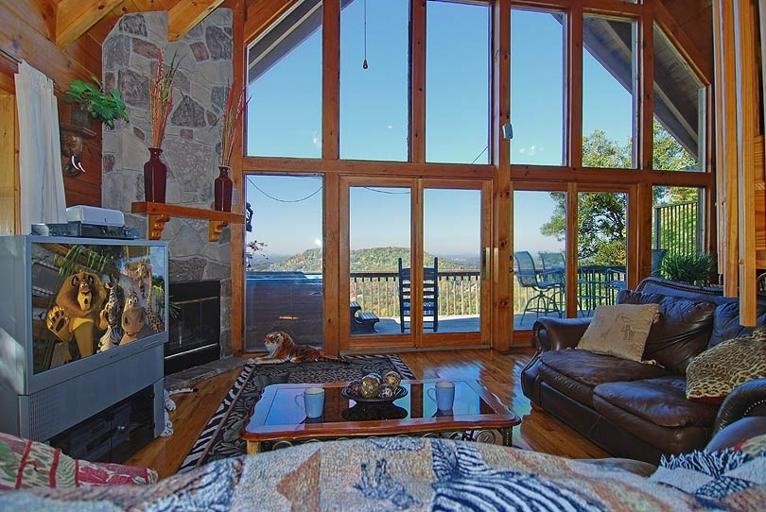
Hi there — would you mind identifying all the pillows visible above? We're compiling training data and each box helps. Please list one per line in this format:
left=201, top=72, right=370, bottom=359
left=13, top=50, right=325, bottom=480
left=573, top=303, right=660, bottom=364
left=684, top=328, right=765, bottom=405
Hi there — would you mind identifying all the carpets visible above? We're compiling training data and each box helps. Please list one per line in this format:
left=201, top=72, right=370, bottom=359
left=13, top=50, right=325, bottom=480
left=177, top=353, right=464, bottom=474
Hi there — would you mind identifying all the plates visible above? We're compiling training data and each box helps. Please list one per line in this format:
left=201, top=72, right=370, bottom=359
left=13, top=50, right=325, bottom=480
left=340, top=384, right=408, bottom=405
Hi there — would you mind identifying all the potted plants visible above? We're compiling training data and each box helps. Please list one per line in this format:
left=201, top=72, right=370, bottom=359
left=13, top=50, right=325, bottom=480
left=63, top=75, right=129, bottom=132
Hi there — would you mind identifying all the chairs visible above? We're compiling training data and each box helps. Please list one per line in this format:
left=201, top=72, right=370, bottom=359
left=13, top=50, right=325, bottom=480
left=513, top=249, right=670, bottom=326
left=397, top=256, right=440, bottom=331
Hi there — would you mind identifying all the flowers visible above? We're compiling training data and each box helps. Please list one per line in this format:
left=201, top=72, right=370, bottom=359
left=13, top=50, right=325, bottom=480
left=218, top=77, right=253, bottom=166
left=148, top=45, right=188, bottom=149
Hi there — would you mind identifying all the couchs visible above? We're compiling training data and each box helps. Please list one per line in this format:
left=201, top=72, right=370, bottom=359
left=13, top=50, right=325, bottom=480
left=521, top=276, right=765, bottom=466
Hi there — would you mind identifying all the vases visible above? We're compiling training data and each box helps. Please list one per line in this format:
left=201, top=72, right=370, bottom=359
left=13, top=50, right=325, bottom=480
left=144, top=149, right=167, bottom=202
left=214, top=167, right=233, bottom=212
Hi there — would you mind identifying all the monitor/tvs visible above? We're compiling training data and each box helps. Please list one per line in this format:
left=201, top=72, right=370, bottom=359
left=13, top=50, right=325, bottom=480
left=0, top=235, right=169, bottom=397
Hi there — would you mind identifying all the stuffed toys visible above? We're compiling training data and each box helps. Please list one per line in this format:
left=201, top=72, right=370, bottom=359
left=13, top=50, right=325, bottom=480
left=252, top=330, right=351, bottom=369
left=158, top=387, right=199, bottom=437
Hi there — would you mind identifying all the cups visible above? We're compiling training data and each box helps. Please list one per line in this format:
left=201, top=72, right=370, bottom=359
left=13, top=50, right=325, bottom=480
left=294, top=388, right=325, bottom=419
left=427, top=380, right=456, bottom=411
